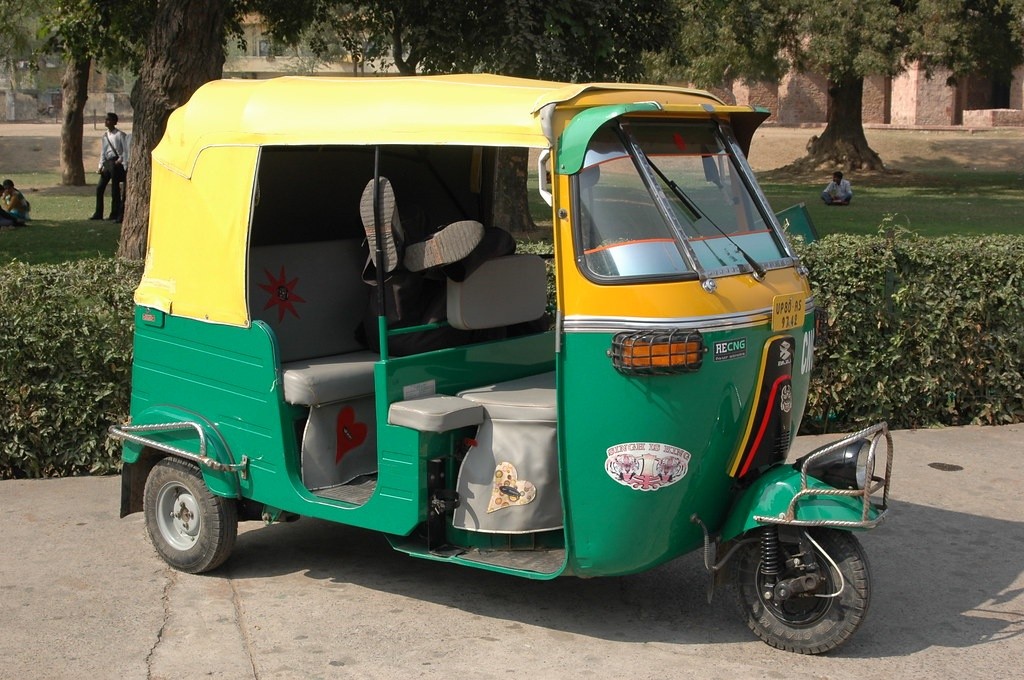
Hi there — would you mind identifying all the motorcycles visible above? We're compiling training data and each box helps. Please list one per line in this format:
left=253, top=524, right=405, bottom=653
left=108, top=72, right=896, bottom=654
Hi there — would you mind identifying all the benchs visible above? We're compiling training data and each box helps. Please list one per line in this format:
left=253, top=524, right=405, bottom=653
left=451, top=368, right=563, bottom=534
left=250, top=239, right=397, bottom=492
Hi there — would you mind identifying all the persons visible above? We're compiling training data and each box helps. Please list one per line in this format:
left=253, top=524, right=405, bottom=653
left=0, top=179, right=30, bottom=226
left=89, top=113, right=132, bottom=223
left=821, top=171, right=852, bottom=205
left=358, top=177, right=548, bottom=355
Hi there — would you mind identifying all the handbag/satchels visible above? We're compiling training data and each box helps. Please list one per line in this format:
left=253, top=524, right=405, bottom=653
left=115, top=165, right=125, bottom=182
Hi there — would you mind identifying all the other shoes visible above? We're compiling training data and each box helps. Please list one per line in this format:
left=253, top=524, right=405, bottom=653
left=109, top=214, right=118, bottom=219
left=359, top=177, right=398, bottom=273
left=404, top=220, right=485, bottom=272
left=90, top=215, right=103, bottom=220
